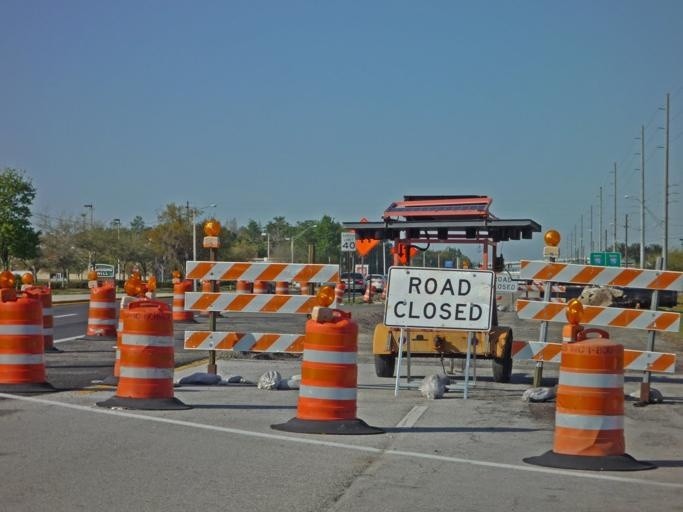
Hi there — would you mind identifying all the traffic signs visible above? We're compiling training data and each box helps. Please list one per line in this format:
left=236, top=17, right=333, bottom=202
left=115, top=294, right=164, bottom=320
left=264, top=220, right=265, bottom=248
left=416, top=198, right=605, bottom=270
left=94, top=264, right=113, bottom=270
left=94, top=269, right=115, bottom=278
left=339, top=230, right=356, bottom=253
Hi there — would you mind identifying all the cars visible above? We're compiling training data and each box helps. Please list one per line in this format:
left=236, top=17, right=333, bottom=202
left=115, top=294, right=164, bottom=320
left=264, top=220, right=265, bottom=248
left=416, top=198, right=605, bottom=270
left=247, top=280, right=275, bottom=295
left=363, top=272, right=386, bottom=294
left=503, top=260, right=680, bottom=310
left=337, top=269, right=366, bottom=294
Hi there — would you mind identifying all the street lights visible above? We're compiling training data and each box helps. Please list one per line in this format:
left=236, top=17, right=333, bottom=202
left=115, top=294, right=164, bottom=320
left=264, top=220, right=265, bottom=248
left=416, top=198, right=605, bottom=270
left=81, top=204, right=92, bottom=227
left=111, top=218, right=121, bottom=281
left=565, top=193, right=668, bottom=270
left=80, top=213, right=86, bottom=230
left=202, top=218, right=224, bottom=376
left=282, top=224, right=318, bottom=263
left=259, top=232, right=270, bottom=261
left=192, top=203, right=216, bottom=261
